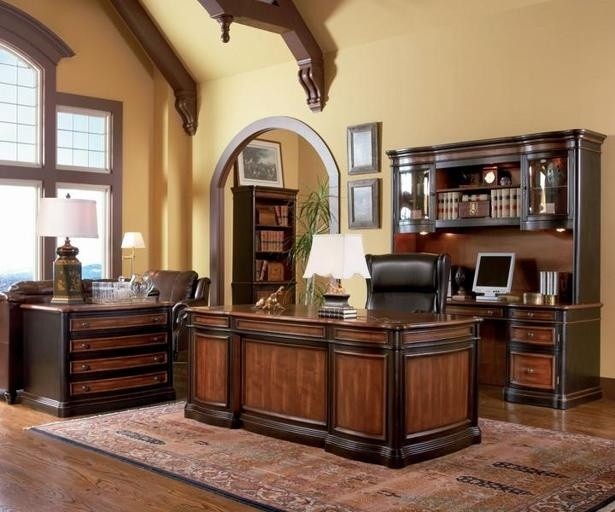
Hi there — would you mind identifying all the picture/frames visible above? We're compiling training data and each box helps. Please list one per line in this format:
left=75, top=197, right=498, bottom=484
left=237, top=138, right=285, bottom=189
left=347, top=122, right=380, bottom=175
left=347, top=178, right=380, bottom=229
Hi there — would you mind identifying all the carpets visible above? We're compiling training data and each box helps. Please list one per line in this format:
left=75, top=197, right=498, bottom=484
left=21, top=400, right=615, bottom=511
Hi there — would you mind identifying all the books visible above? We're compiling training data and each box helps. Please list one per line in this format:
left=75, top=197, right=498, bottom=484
left=319, top=306, right=357, bottom=314
left=316, top=312, right=358, bottom=319
left=539, top=270, right=568, bottom=298
left=431, top=185, right=523, bottom=219
left=255, top=201, right=290, bottom=281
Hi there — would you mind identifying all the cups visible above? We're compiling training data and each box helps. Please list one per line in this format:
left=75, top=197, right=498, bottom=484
left=91, top=271, right=154, bottom=306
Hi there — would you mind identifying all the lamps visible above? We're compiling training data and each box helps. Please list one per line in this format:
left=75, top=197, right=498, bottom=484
left=120, top=232, right=146, bottom=283
left=35, top=193, right=98, bottom=305
left=301, top=233, right=373, bottom=319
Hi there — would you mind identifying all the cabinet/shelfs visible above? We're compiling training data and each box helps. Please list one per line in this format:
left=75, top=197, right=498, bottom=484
left=19, top=298, right=177, bottom=418
left=230, top=185, right=299, bottom=305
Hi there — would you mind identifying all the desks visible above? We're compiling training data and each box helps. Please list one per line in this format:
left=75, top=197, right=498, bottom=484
left=183, top=303, right=485, bottom=469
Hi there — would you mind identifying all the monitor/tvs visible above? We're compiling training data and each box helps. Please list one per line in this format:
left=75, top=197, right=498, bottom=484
left=472, top=251, right=515, bottom=301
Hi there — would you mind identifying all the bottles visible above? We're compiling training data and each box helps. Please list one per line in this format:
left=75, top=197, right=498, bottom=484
left=542, top=162, right=561, bottom=187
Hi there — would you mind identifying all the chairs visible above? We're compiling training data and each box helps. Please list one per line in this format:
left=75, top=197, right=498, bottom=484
left=364, top=252, right=452, bottom=314
left=141, top=269, right=212, bottom=364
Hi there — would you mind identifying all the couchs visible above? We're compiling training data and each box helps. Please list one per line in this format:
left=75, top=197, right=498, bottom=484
left=0, top=279, right=160, bottom=405
